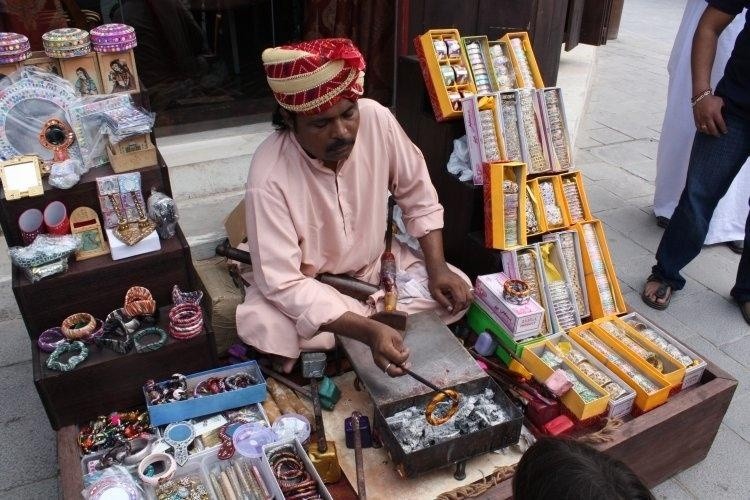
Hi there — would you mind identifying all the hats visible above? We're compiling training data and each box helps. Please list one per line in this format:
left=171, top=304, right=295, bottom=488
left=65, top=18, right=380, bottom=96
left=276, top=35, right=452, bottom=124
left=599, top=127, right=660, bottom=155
left=256, top=35, right=368, bottom=115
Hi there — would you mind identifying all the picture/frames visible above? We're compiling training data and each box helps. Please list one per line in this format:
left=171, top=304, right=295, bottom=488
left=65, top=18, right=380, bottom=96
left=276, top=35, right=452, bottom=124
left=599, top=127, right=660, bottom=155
left=1, top=46, right=142, bottom=171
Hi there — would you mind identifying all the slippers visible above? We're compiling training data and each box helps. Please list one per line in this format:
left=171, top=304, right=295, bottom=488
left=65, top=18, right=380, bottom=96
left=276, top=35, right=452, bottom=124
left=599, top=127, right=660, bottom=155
left=640, top=272, right=673, bottom=310
left=737, top=299, right=750, bottom=325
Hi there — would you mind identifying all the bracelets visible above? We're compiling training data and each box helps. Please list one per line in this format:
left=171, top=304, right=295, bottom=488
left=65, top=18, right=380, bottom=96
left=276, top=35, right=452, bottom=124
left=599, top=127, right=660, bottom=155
left=503, top=178, right=584, bottom=247
left=434, top=36, right=539, bottom=110
left=423, top=389, right=459, bottom=425
left=32, top=282, right=204, bottom=371
left=480, top=90, right=571, bottom=171
left=59, top=374, right=333, bottom=500
left=516, top=223, right=618, bottom=333
left=536, top=313, right=699, bottom=406
left=691, top=88, right=712, bottom=108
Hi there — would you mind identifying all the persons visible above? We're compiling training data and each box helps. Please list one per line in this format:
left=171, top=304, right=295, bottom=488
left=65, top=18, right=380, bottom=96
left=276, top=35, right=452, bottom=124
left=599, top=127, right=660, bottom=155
left=234, top=39, right=475, bottom=378
left=511, top=434, right=655, bottom=500
left=76, top=67, right=98, bottom=96
left=640, top=0, right=749, bottom=323
left=651, top=0, right=749, bottom=253
left=108, top=58, right=136, bottom=93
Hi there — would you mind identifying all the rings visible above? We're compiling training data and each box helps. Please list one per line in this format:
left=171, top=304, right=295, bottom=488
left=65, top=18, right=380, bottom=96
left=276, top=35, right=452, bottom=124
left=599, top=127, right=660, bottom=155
left=381, top=362, right=392, bottom=371
left=470, top=288, right=475, bottom=291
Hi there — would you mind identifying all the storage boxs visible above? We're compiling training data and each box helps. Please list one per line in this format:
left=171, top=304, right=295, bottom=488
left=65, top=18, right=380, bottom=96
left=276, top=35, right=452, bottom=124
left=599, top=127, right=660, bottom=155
left=81, top=361, right=334, bottom=500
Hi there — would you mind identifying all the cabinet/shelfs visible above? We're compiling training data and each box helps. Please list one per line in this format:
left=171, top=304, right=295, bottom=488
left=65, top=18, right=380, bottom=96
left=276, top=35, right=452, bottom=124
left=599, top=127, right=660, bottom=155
left=393, top=51, right=507, bottom=290
left=0, top=105, right=217, bottom=431
